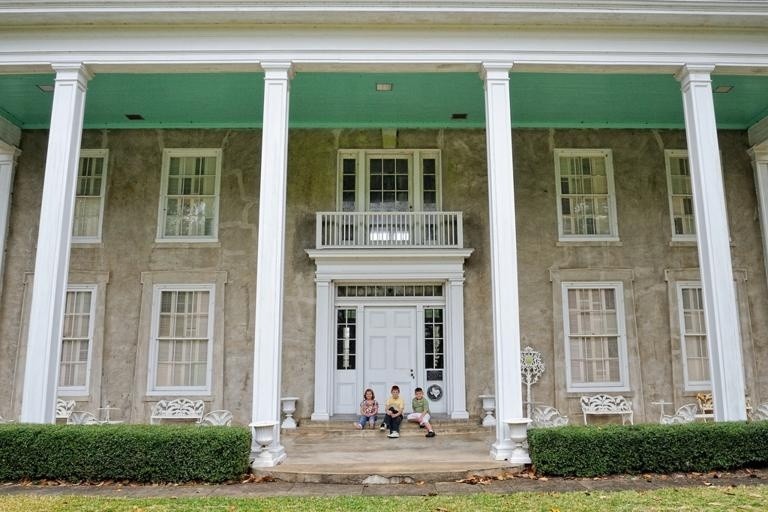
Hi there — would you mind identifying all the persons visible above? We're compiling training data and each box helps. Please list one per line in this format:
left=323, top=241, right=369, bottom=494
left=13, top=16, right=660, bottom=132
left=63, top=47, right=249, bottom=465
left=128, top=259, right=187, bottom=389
left=379, top=385, right=405, bottom=438
left=406, top=386, right=435, bottom=437
left=353, top=388, right=379, bottom=430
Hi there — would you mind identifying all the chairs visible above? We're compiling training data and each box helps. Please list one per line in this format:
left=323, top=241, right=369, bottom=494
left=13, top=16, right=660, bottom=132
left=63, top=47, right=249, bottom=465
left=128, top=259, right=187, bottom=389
left=532, top=405, right=568, bottom=430
left=662, top=403, right=698, bottom=426
left=197, top=410, right=235, bottom=431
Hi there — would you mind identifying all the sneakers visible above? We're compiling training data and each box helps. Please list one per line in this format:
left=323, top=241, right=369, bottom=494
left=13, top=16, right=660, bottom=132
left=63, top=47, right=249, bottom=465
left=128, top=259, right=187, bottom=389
left=425, top=431, right=435, bottom=437
left=379, top=422, right=387, bottom=430
left=387, top=431, right=400, bottom=437
left=353, top=422, right=363, bottom=430
left=370, top=418, right=374, bottom=429
left=420, top=423, right=425, bottom=428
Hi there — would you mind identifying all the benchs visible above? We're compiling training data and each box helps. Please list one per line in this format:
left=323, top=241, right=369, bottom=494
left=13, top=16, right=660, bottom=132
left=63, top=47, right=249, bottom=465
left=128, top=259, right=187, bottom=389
left=581, top=395, right=636, bottom=424
left=56, top=399, right=75, bottom=423
left=150, top=397, right=202, bottom=426
left=695, top=393, right=755, bottom=422
